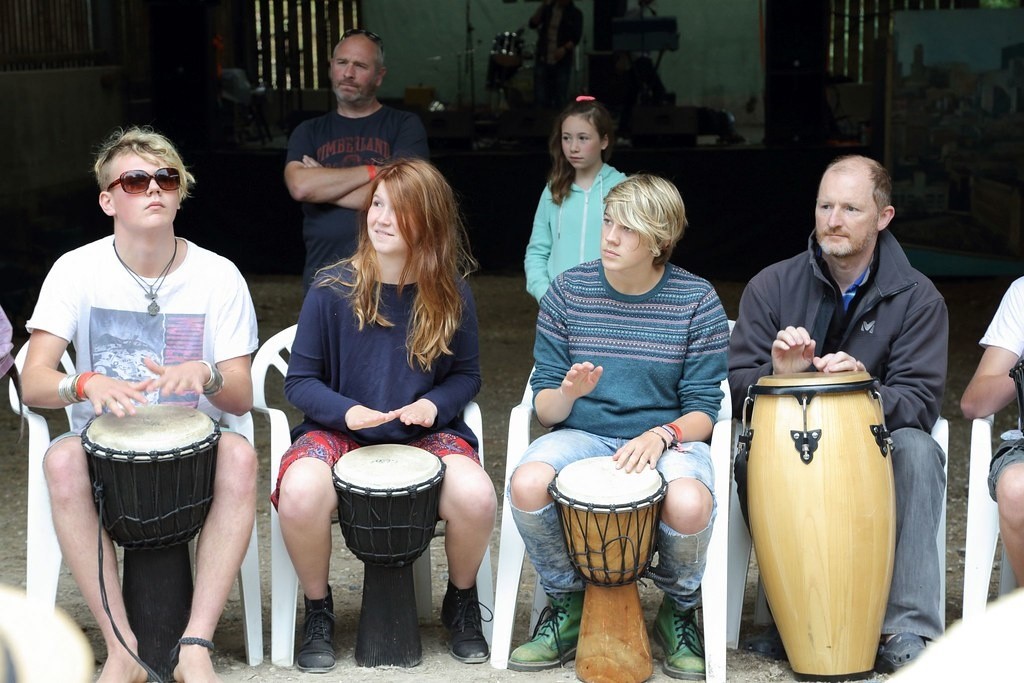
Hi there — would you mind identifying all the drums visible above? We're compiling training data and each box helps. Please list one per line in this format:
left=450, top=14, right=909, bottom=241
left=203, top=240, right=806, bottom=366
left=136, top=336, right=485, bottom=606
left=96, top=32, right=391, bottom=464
left=80, top=402, right=223, bottom=682
left=491, top=31, right=521, bottom=67
left=548, top=456, right=669, bottom=682
left=329, top=442, right=448, bottom=670
left=743, top=369, right=900, bottom=682
left=1008, top=349, right=1024, bottom=434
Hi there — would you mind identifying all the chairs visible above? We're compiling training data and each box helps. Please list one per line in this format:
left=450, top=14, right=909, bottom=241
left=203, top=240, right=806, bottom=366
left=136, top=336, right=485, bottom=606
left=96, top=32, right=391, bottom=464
left=9, top=340, right=263, bottom=666
left=963, top=413, right=1021, bottom=620
left=251, top=323, right=494, bottom=666
left=727, top=320, right=948, bottom=651
left=490, top=320, right=732, bottom=682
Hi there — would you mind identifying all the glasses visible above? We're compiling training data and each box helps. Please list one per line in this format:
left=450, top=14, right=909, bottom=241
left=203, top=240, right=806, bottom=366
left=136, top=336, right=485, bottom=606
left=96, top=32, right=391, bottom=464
left=107, top=167, right=180, bottom=194
left=339, top=29, right=384, bottom=57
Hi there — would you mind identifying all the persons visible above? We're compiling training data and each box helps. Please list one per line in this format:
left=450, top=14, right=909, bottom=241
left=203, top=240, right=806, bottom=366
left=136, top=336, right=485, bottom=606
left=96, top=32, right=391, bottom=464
left=283, top=29, right=430, bottom=293
left=19, top=126, right=258, bottom=683
left=527, top=0, right=584, bottom=92
left=508, top=173, right=728, bottom=680
left=727, top=157, right=947, bottom=671
left=270, top=155, right=497, bottom=675
left=522, top=95, right=629, bottom=303
left=960, top=276, right=1024, bottom=588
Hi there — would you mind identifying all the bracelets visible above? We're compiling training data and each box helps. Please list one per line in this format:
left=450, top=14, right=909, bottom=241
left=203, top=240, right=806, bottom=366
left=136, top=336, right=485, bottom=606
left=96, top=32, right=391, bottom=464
left=649, top=424, right=693, bottom=452
left=196, top=358, right=225, bottom=397
left=58, top=371, right=102, bottom=404
left=367, top=166, right=377, bottom=180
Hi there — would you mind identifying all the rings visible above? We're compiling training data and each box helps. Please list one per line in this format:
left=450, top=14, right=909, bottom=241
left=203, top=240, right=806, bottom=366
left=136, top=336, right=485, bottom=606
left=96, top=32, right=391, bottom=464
left=105, top=397, right=115, bottom=407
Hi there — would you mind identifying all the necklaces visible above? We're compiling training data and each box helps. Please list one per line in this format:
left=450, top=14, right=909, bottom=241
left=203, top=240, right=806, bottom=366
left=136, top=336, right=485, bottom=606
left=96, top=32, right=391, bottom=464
left=112, top=237, right=176, bottom=317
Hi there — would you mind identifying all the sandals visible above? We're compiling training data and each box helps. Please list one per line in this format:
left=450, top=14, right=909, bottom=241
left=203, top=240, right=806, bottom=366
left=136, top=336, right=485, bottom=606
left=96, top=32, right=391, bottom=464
left=744, top=626, right=788, bottom=663
left=877, top=632, right=926, bottom=674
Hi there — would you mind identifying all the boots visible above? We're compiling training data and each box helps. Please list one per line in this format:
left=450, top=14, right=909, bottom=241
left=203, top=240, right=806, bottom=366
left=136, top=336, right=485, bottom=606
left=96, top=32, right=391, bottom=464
left=440, top=578, right=493, bottom=663
left=296, top=584, right=337, bottom=672
left=653, top=591, right=706, bottom=680
left=507, top=589, right=585, bottom=671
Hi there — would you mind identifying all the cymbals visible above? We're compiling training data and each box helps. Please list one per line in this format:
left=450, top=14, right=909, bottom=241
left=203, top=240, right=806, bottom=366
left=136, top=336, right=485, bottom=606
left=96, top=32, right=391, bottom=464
left=575, top=49, right=613, bottom=56
